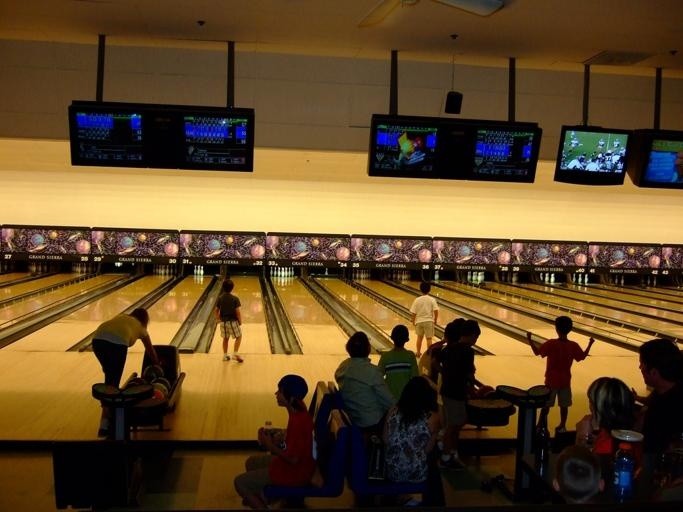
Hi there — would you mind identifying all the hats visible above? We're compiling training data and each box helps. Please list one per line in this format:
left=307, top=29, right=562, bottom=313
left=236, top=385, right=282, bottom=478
left=279, top=375, right=308, bottom=411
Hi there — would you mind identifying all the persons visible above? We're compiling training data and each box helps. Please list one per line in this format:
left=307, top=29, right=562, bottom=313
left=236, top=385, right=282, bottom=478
left=526, top=316, right=593, bottom=430
left=233, top=375, right=314, bottom=511
left=91, top=308, right=161, bottom=437
left=334, top=331, right=398, bottom=441
left=214, top=280, right=244, bottom=362
left=559, top=131, right=626, bottom=174
left=378, top=324, right=418, bottom=407
left=424, top=318, right=465, bottom=454
left=383, top=375, right=441, bottom=483
left=436, top=320, right=494, bottom=471
left=550, top=337, right=682, bottom=511
left=410, top=282, right=438, bottom=358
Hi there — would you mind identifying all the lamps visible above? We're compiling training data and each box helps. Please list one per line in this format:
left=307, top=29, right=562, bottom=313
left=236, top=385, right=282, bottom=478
left=444, top=35, right=463, bottom=114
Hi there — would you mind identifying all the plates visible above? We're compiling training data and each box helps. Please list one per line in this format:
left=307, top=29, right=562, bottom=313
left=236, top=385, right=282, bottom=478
left=611, top=428, right=644, bottom=443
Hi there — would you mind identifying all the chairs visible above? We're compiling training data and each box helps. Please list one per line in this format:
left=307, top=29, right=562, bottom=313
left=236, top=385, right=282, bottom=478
left=259, top=382, right=434, bottom=512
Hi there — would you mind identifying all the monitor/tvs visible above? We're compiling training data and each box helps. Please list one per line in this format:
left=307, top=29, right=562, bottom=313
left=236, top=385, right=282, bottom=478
left=173, top=106, right=254, bottom=172
left=68, top=102, right=152, bottom=169
left=367, top=115, right=446, bottom=178
left=632, top=129, right=683, bottom=188
left=554, top=124, right=628, bottom=184
left=459, top=120, right=536, bottom=184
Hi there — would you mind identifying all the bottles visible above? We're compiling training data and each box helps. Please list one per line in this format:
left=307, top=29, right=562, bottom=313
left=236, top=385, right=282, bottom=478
left=535, top=410, right=550, bottom=464
left=261, top=420, right=273, bottom=437
left=612, top=442, right=637, bottom=505
left=496, top=475, right=506, bottom=491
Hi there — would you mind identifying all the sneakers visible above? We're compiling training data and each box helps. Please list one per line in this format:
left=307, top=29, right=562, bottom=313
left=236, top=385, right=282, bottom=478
left=223, top=354, right=243, bottom=362
left=439, top=452, right=466, bottom=470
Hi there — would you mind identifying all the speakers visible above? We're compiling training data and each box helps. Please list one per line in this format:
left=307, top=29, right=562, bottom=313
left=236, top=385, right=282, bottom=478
left=445, top=91, right=462, bottom=114
